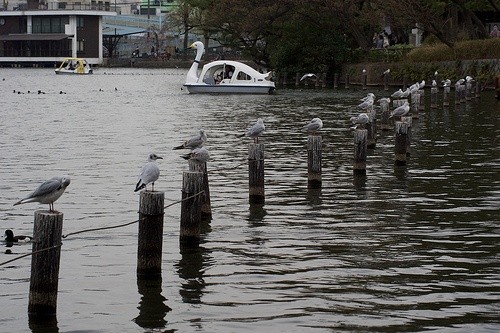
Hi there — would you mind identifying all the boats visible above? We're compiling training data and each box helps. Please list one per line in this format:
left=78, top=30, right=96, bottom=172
left=182, top=40, right=276, bottom=95
left=54, top=58, right=94, bottom=75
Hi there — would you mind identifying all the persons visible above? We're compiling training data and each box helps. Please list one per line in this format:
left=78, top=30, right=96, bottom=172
left=372, top=29, right=397, bottom=50
left=69, top=62, right=79, bottom=70
left=214, top=68, right=233, bottom=85
left=135, top=45, right=140, bottom=57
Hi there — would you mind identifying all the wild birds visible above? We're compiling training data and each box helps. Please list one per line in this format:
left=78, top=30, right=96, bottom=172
left=134, top=154, right=164, bottom=192
left=3, top=229, right=35, bottom=244
left=238, top=117, right=266, bottom=143
left=383, top=68, right=392, bottom=75
left=358, top=69, right=473, bottom=109
left=302, top=117, right=323, bottom=135
left=300, top=73, right=319, bottom=80
left=180, top=150, right=211, bottom=161
left=173, top=130, right=208, bottom=151
left=350, top=113, right=370, bottom=126
left=13, top=176, right=71, bottom=214
left=390, top=101, right=410, bottom=118
